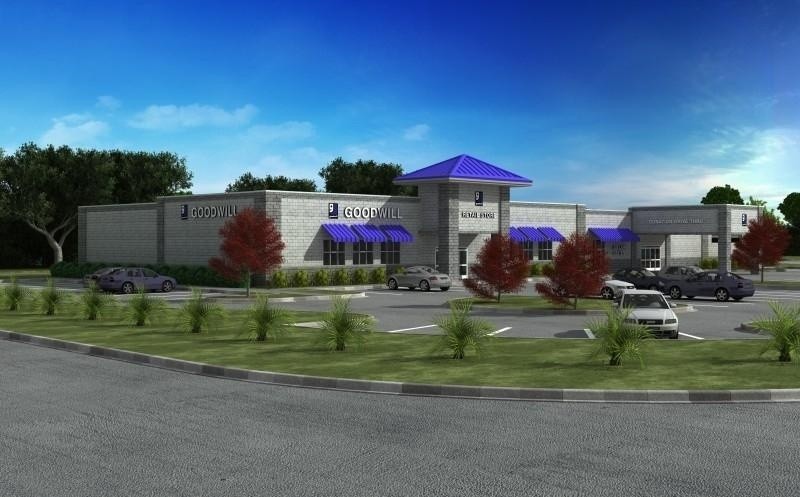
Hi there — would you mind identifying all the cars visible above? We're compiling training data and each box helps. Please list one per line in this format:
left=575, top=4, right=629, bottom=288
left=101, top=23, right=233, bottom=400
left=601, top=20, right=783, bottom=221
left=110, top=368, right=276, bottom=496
left=666, top=269, right=757, bottom=304
left=101, top=267, right=180, bottom=297
left=386, top=265, right=454, bottom=293
left=84, top=267, right=122, bottom=290
left=553, top=258, right=706, bottom=302
left=611, top=288, right=681, bottom=341
